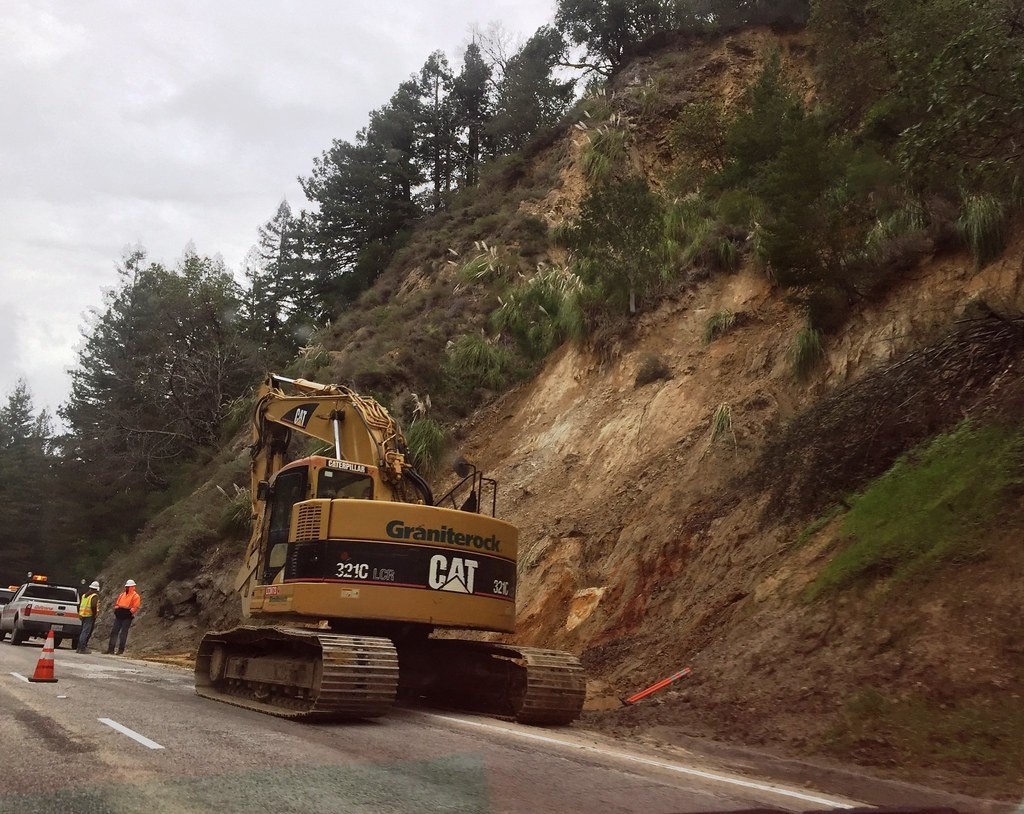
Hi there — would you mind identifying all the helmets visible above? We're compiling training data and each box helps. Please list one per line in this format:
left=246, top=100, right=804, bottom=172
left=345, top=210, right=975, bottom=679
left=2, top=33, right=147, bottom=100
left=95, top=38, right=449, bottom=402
left=89, top=581, right=100, bottom=592
left=125, top=579, right=137, bottom=586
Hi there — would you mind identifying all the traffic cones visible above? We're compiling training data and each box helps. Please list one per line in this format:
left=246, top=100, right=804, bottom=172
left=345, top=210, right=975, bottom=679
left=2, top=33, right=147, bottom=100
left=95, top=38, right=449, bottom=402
left=26, top=629, right=60, bottom=684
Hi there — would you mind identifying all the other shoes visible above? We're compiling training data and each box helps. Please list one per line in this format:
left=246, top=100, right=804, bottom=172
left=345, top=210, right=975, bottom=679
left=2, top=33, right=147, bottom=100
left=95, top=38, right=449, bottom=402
left=116, top=651, right=123, bottom=655
left=101, top=650, right=114, bottom=654
left=77, top=649, right=92, bottom=654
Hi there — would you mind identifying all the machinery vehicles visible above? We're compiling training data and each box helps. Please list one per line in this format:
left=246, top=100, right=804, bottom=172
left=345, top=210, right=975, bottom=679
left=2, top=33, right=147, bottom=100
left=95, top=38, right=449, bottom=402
left=190, top=370, right=590, bottom=733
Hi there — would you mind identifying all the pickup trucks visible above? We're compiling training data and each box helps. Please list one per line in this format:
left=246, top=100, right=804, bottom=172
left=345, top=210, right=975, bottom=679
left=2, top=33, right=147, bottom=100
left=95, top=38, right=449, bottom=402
left=0, top=585, right=21, bottom=620
left=0, top=573, right=84, bottom=650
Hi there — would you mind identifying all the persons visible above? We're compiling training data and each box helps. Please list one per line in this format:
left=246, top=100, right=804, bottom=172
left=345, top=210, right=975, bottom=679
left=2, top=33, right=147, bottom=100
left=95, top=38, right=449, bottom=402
left=75, top=581, right=100, bottom=654
left=101, top=579, right=140, bottom=655
